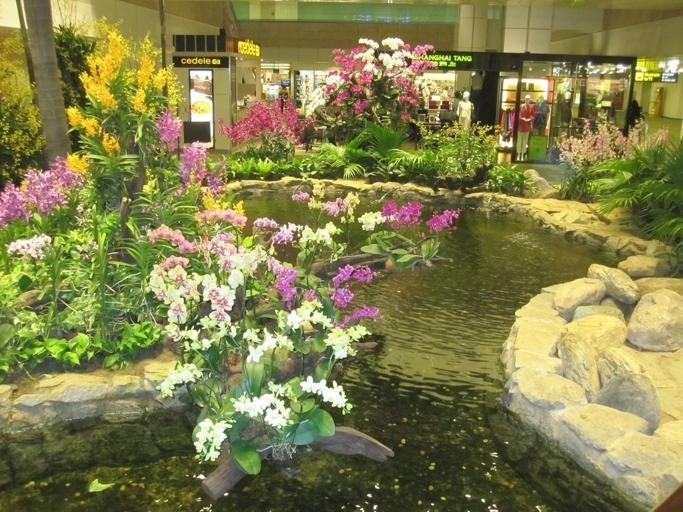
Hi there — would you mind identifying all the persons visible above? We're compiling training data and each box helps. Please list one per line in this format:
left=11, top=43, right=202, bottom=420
left=516, top=95, right=536, bottom=162
left=456, top=91, right=476, bottom=129
left=560, top=92, right=572, bottom=125
left=533, top=96, right=550, bottom=126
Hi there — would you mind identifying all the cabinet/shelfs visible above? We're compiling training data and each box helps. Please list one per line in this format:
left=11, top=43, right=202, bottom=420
left=501, top=77, right=555, bottom=105
